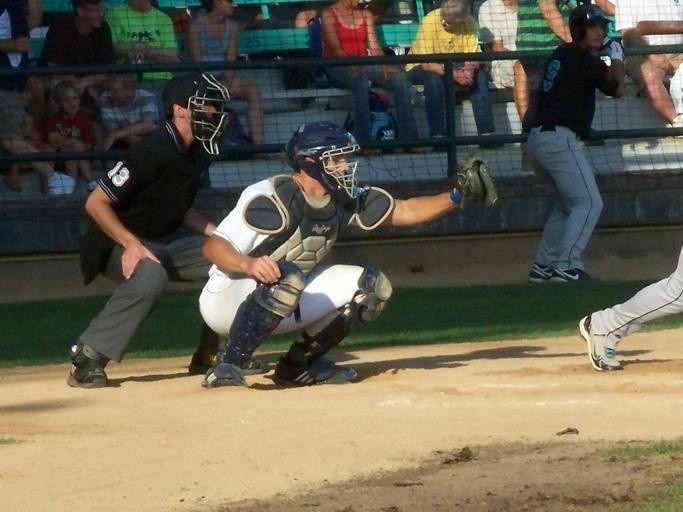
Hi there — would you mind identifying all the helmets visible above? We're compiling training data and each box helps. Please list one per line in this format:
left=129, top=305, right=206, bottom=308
left=568, top=4, right=610, bottom=45
left=286, top=121, right=360, bottom=206
left=162, top=74, right=229, bottom=155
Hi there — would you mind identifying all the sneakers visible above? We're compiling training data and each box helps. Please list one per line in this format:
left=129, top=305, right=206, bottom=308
left=577, top=314, right=622, bottom=370
left=529, top=264, right=588, bottom=281
left=189, top=349, right=357, bottom=388
left=68, top=358, right=107, bottom=388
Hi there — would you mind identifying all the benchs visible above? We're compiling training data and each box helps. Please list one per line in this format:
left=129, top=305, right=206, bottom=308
left=26, top=0, right=483, bottom=111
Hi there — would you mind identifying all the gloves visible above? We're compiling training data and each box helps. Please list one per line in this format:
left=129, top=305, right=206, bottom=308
left=605, top=40, right=622, bottom=61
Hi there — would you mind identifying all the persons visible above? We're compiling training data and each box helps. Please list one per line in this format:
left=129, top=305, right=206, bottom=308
left=1, top=2, right=281, bottom=194
left=198, top=120, right=461, bottom=389
left=238, top=2, right=683, bottom=155
left=524, top=3, right=626, bottom=284
left=578, top=246, right=683, bottom=373
left=67, top=70, right=270, bottom=389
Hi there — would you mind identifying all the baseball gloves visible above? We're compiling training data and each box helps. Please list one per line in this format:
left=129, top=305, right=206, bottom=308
left=451, top=158, right=500, bottom=214
